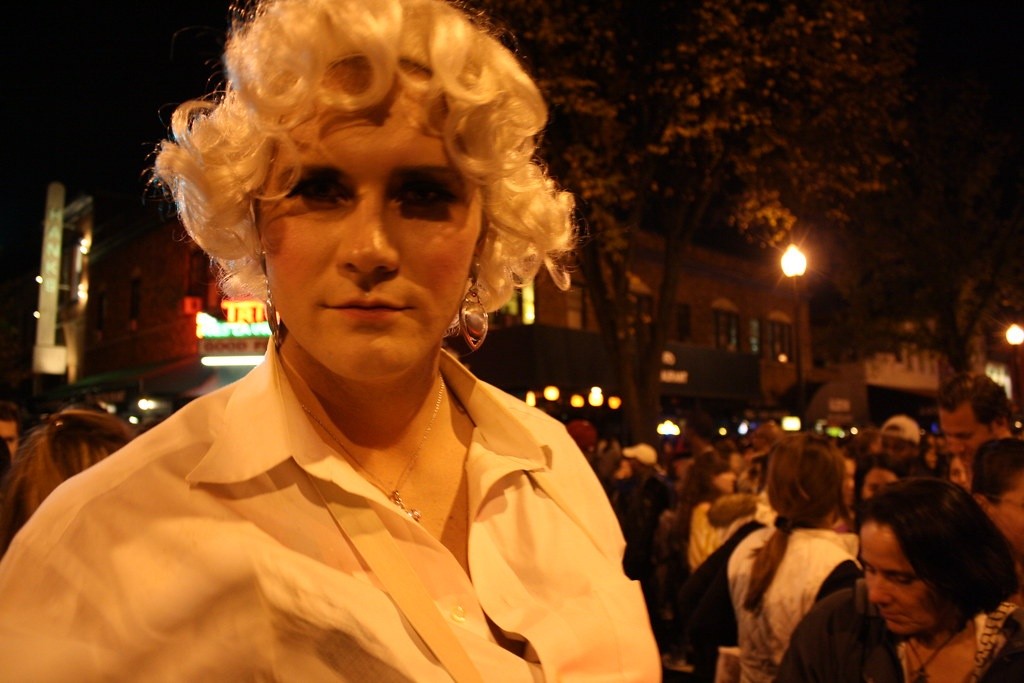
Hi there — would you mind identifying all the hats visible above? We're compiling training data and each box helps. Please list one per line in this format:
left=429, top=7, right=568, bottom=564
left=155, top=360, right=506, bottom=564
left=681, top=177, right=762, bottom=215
left=623, top=444, right=657, bottom=466
left=565, top=420, right=594, bottom=449
left=881, top=415, right=920, bottom=443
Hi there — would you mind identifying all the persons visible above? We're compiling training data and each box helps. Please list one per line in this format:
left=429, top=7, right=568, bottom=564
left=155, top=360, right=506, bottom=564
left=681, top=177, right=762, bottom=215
left=0, top=0, right=665, bottom=682
left=0, top=397, right=144, bottom=563
left=568, top=369, right=1024, bottom=683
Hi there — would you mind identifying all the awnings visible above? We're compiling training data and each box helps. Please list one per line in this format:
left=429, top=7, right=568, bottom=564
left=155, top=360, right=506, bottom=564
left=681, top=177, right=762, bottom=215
left=42, top=358, right=150, bottom=395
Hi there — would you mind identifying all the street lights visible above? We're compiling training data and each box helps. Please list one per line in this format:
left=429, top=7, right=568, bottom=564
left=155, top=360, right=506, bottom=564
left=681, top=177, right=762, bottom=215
left=780, top=243, right=807, bottom=429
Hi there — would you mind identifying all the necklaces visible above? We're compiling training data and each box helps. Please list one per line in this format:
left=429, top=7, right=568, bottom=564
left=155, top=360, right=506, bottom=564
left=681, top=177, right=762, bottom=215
left=906, top=627, right=958, bottom=683
left=301, top=369, right=445, bottom=523
